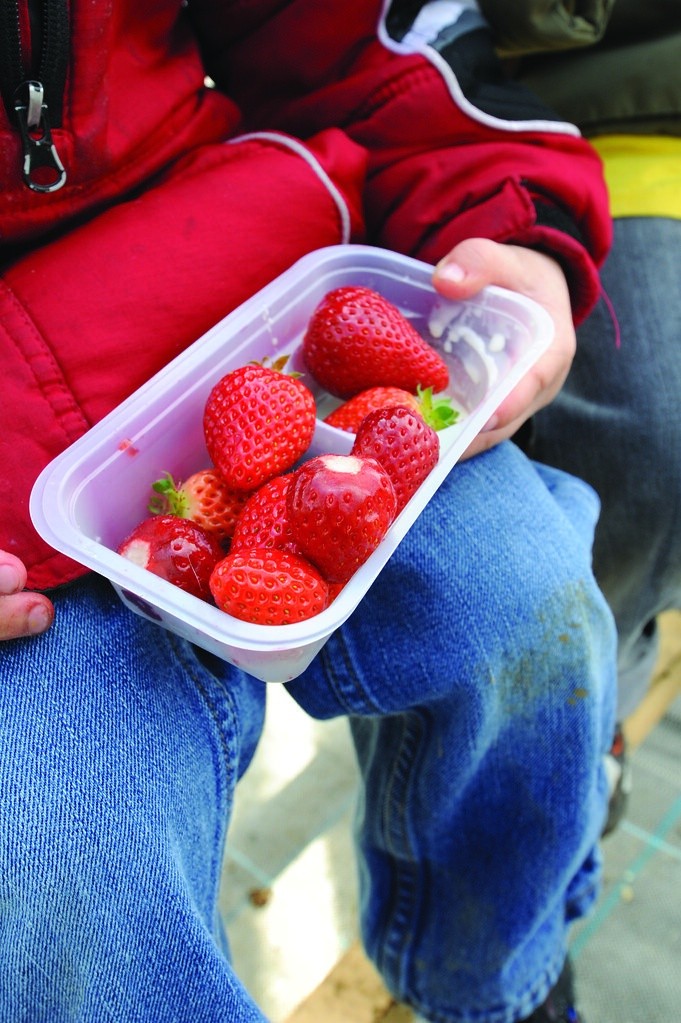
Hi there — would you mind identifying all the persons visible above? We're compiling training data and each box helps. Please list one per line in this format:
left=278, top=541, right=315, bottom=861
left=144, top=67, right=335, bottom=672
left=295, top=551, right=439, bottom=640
left=0, top=0, right=681, bottom=1023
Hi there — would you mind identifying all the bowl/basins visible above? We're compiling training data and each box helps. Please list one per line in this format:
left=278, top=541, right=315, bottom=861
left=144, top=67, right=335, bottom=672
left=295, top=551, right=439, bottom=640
left=26, top=242, right=560, bottom=685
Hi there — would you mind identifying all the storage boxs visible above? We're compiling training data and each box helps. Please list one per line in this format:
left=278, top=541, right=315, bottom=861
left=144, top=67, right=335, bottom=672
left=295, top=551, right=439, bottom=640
left=27, top=248, right=551, bottom=681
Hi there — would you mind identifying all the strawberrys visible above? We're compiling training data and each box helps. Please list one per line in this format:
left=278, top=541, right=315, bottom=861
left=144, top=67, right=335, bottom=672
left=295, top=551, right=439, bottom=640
left=301, top=285, right=449, bottom=397
left=117, top=355, right=461, bottom=626
